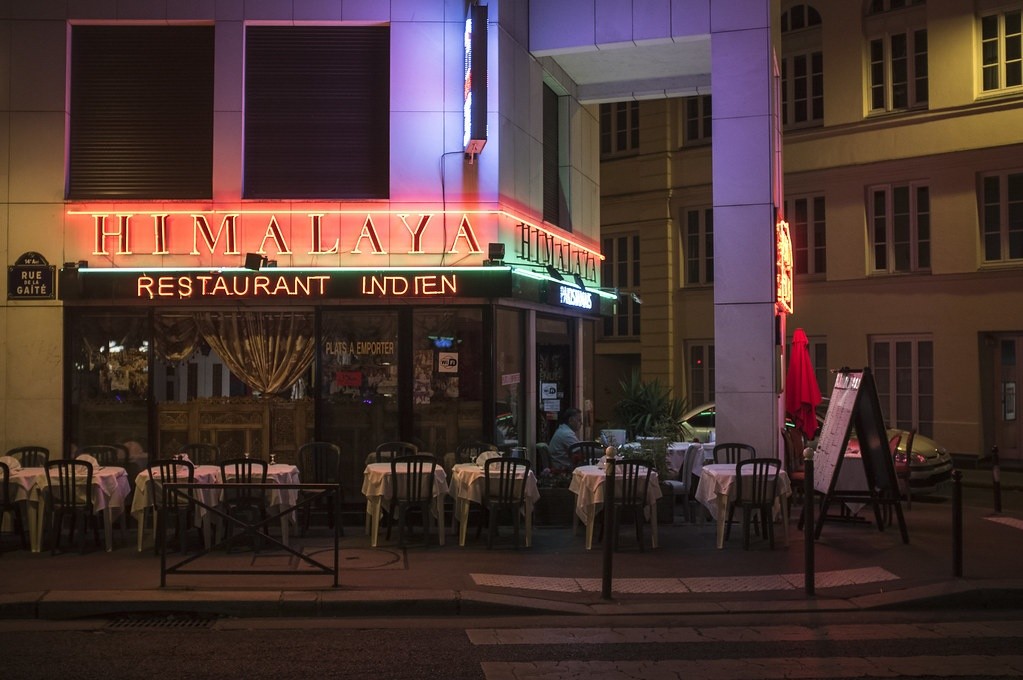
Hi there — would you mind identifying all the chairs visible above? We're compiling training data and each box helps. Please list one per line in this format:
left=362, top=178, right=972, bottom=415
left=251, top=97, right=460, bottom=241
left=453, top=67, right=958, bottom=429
left=725, top=459, right=781, bottom=550
left=712, top=443, right=756, bottom=465
left=147, top=460, right=203, bottom=555
left=780, top=423, right=920, bottom=528
left=220, top=458, right=269, bottom=551
left=569, top=441, right=701, bottom=551
left=177, top=444, right=219, bottom=466
left=536, top=443, right=552, bottom=472
left=454, top=439, right=500, bottom=464
left=0, top=445, right=118, bottom=555
left=297, top=442, right=341, bottom=536
left=476, top=457, right=532, bottom=547
left=375, top=441, right=420, bottom=464
left=385, top=454, right=437, bottom=547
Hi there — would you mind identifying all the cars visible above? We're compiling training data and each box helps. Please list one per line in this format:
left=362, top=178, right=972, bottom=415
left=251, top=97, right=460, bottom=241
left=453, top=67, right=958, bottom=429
left=674, top=402, right=954, bottom=496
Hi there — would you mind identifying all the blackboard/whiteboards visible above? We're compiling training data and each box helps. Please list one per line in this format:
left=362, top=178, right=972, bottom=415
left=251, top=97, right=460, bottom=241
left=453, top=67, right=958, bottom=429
left=813, top=366, right=902, bottom=502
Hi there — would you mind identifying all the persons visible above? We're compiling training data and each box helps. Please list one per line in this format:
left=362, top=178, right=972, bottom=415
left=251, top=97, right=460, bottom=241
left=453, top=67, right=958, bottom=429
left=548, top=407, right=604, bottom=475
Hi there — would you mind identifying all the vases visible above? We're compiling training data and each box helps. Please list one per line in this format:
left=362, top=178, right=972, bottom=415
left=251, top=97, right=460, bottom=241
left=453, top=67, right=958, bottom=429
left=533, top=488, right=574, bottom=528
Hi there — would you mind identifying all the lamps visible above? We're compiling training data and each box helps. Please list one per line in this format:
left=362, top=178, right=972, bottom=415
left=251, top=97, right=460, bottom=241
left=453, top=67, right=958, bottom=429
left=585, top=286, right=624, bottom=305
left=546, top=265, right=564, bottom=281
left=608, top=290, right=644, bottom=305
left=487, top=242, right=506, bottom=264
left=382, top=356, right=390, bottom=365
left=574, top=273, right=585, bottom=291
left=244, top=252, right=268, bottom=271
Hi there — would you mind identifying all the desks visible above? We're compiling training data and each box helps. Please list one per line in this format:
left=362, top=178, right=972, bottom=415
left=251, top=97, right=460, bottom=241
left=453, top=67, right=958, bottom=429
left=694, top=463, right=792, bottom=549
left=29, top=465, right=130, bottom=552
left=818, top=452, right=881, bottom=525
left=446, top=462, right=540, bottom=548
left=622, top=442, right=703, bottom=479
left=214, top=462, right=299, bottom=547
left=0, top=466, right=46, bottom=552
left=130, top=464, right=224, bottom=552
left=569, top=464, right=663, bottom=550
left=362, top=462, right=449, bottom=547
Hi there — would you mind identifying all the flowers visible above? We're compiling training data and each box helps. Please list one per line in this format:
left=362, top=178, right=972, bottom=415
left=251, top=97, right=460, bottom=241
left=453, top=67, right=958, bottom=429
left=537, top=465, right=575, bottom=488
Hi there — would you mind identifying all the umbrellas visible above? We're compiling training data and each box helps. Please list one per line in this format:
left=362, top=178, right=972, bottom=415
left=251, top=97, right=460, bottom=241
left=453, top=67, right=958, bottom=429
left=786, top=327, right=823, bottom=448
